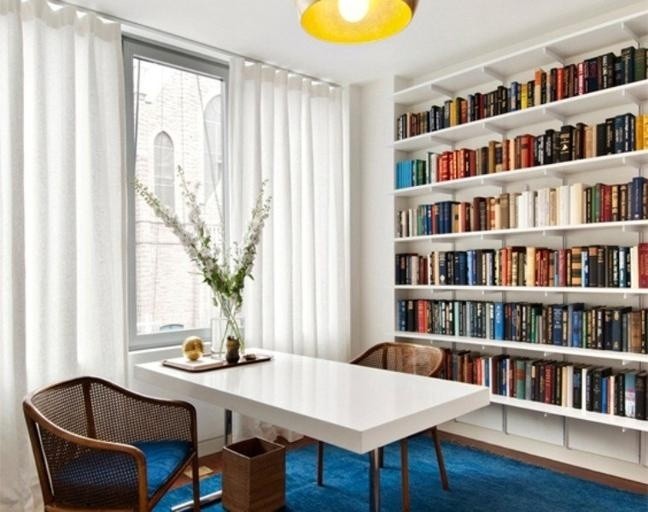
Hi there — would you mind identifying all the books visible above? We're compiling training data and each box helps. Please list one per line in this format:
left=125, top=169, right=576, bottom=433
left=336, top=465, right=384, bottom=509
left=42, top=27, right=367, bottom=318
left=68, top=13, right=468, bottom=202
left=394, top=43, right=647, bottom=422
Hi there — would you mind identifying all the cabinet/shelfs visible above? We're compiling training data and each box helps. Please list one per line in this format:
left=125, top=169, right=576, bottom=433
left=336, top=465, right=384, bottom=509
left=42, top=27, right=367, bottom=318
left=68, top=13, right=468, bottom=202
left=394, top=9, right=648, bottom=497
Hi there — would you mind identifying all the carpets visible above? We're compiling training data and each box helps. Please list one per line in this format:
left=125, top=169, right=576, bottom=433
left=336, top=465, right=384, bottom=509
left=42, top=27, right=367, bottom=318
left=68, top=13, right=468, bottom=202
left=153, top=434, right=647, bottom=512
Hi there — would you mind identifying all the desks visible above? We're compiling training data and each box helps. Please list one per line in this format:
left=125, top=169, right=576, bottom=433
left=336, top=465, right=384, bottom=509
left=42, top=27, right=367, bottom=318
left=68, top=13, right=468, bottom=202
left=134, top=345, right=490, bottom=512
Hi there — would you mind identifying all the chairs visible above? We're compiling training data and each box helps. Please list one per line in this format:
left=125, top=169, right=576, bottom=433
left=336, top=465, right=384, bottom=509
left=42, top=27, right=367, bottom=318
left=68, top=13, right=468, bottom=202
left=22, top=376, right=200, bottom=512
left=317, top=344, right=449, bottom=511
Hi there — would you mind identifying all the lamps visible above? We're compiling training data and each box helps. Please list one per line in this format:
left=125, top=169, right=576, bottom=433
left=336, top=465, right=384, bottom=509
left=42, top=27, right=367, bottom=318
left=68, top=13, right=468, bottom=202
left=298, top=1, right=420, bottom=45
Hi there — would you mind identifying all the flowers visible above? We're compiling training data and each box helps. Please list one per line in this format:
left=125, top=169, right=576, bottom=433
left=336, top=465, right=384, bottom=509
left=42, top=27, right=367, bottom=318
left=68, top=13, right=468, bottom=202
left=130, top=164, right=273, bottom=351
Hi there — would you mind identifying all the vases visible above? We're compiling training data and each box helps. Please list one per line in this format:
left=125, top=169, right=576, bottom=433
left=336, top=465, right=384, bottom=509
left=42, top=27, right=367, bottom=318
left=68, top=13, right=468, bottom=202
left=213, top=297, right=248, bottom=356
